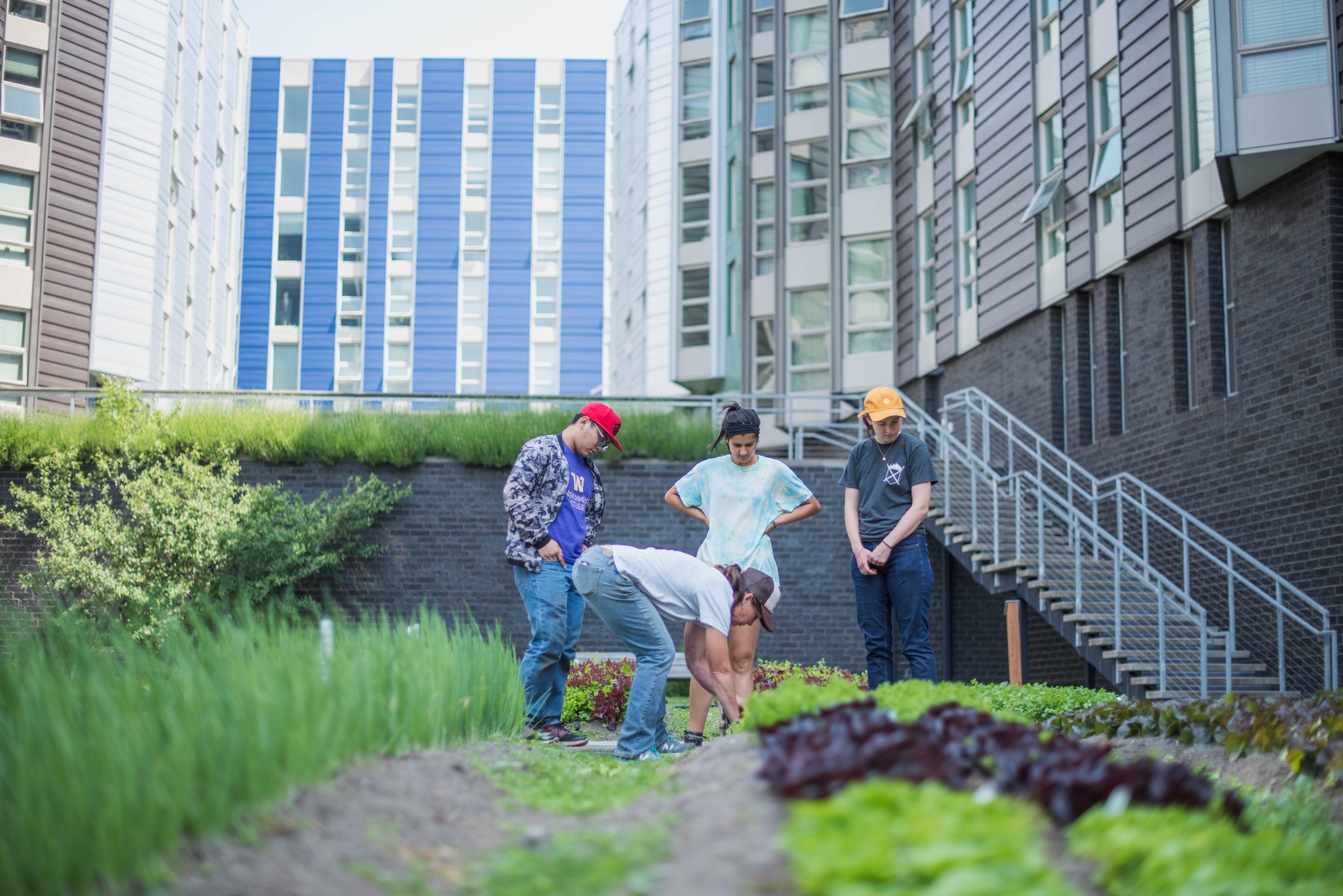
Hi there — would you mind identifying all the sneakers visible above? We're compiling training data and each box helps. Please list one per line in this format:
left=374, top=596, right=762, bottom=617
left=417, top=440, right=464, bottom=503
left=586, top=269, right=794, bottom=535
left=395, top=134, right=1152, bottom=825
left=521, top=721, right=559, bottom=744
left=543, top=721, right=589, bottom=746
left=615, top=744, right=662, bottom=763
left=656, top=732, right=688, bottom=755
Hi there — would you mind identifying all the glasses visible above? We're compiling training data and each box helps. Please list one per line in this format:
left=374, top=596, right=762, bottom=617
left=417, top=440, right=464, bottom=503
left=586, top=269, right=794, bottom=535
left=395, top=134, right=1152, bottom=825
left=593, top=422, right=609, bottom=453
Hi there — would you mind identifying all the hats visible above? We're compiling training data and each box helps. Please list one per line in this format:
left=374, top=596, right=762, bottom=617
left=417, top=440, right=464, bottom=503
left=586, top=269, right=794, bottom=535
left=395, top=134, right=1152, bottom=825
left=857, top=386, right=906, bottom=421
left=580, top=403, right=623, bottom=452
left=741, top=567, right=781, bottom=632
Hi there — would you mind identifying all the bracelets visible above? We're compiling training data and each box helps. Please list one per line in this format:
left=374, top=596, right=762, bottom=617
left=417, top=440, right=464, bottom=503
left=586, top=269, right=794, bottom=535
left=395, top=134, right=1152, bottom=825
left=855, top=547, right=864, bottom=558
left=771, top=521, right=776, bottom=528
left=882, top=540, right=892, bottom=549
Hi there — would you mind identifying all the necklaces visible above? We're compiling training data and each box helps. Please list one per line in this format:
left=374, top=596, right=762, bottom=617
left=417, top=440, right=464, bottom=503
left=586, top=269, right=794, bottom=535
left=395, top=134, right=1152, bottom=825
left=875, top=436, right=894, bottom=461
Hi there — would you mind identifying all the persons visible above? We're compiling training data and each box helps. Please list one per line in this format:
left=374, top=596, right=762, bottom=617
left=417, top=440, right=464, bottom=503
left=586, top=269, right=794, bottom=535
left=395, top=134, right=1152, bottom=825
left=572, top=544, right=781, bottom=767
left=664, top=399, right=821, bottom=748
left=838, top=386, right=937, bottom=690
left=503, top=402, right=623, bottom=747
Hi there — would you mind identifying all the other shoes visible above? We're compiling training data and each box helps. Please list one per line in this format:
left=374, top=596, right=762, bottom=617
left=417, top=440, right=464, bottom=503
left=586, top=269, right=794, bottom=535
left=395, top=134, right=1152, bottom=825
left=683, top=730, right=703, bottom=747
